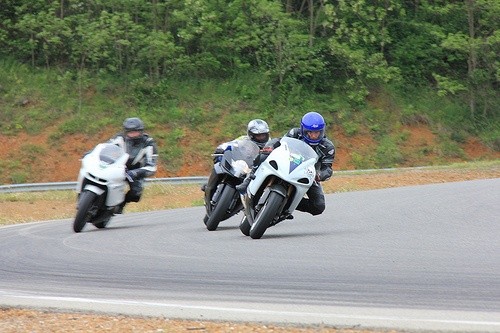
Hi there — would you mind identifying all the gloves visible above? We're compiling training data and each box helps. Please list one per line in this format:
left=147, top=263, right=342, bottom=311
left=129, top=169, right=145, bottom=180
left=81, top=150, right=91, bottom=157
left=317, top=169, right=330, bottom=181
left=216, top=149, right=224, bottom=154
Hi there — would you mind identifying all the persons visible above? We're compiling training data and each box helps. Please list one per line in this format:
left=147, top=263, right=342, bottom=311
left=81, top=117, right=159, bottom=214
left=236, top=112, right=335, bottom=215
left=201, top=119, right=271, bottom=191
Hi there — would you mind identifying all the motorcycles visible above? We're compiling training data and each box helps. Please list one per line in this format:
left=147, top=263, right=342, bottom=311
left=238, top=135, right=319, bottom=239
left=72, top=143, right=135, bottom=233
left=201, top=138, right=260, bottom=231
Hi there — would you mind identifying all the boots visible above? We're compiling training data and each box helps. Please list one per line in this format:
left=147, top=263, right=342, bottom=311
left=235, top=178, right=250, bottom=190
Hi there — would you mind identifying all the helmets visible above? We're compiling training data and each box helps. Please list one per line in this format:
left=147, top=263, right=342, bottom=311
left=247, top=119, right=270, bottom=148
left=123, top=118, right=144, bottom=142
left=300, top=112, right=326, bottom=143
